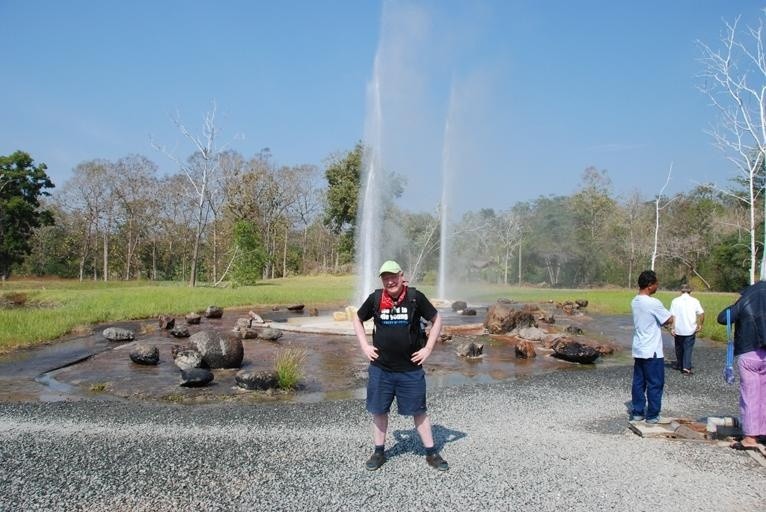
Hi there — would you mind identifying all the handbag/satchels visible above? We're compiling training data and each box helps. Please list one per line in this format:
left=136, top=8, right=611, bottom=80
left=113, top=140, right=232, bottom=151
left=722, top=307, right=735, bottom=384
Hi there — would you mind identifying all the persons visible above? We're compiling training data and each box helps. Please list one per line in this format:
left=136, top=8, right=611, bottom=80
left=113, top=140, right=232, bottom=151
left=351, top=260, right=449, bottom=471
left=669, top=284, right=704, bottom=374
left=717, top=279, right=766, bottom=450
left=631, top=269, right=674, bottom=424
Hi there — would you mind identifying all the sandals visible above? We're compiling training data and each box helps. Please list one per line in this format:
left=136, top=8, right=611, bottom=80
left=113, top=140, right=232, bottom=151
left=683, top=368, right=695, bottom=374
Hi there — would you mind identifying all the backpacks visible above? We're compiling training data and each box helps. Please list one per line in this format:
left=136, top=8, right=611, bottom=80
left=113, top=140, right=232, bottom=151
left=374, top=286, right=427, bottom=349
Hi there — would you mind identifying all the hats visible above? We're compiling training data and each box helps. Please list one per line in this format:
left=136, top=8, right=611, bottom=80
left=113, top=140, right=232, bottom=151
left=378, top=260, right=402, bottom=277
left=680, top=284, right=693, bottom=293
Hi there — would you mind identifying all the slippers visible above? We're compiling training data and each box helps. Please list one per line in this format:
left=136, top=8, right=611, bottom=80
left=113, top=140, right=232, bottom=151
left=730, top=441, right=757, bottom=450
left=646, top=416, right=673, bottom=425
left=365, top=454, right=387, bottom=470
left=426, top=453, right=449, bottom=470
